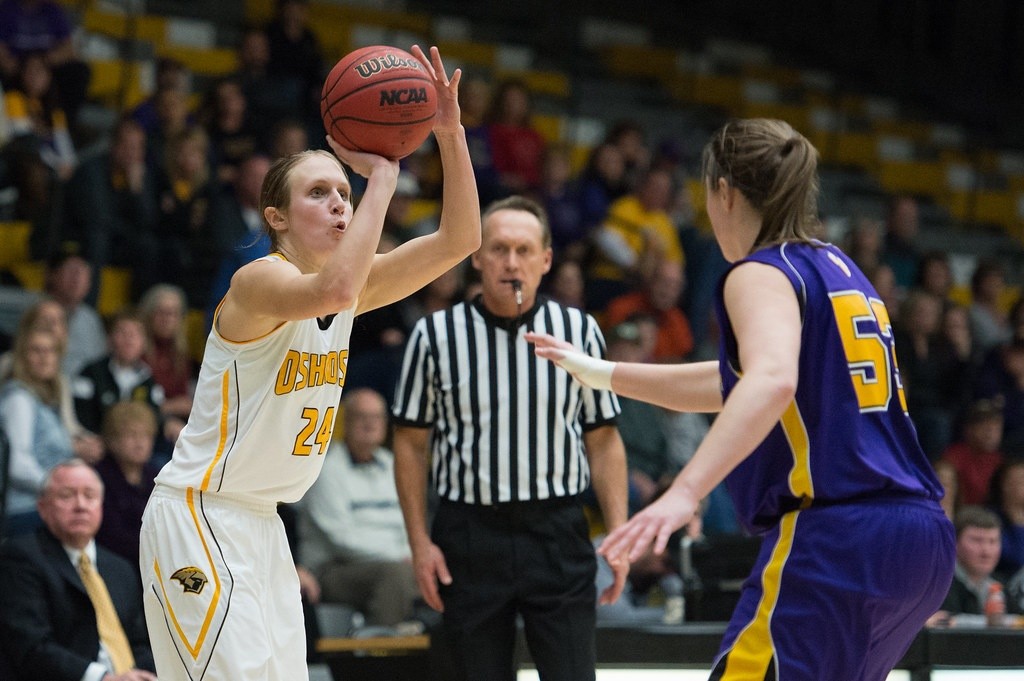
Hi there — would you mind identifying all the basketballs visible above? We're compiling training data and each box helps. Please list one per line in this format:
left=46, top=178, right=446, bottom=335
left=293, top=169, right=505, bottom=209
left=318, top=45, right=437, bottom=160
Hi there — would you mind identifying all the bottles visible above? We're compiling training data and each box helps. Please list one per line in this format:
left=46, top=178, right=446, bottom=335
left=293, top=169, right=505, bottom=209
left=985, top=583, right=1006, bottom=626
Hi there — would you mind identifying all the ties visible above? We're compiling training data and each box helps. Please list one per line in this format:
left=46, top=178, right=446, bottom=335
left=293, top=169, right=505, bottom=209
left=78, top=550, right=136, bottom=676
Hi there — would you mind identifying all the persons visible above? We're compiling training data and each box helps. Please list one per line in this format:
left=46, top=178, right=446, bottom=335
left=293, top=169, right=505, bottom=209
left=0, top=0, right=1024, bottom=681
